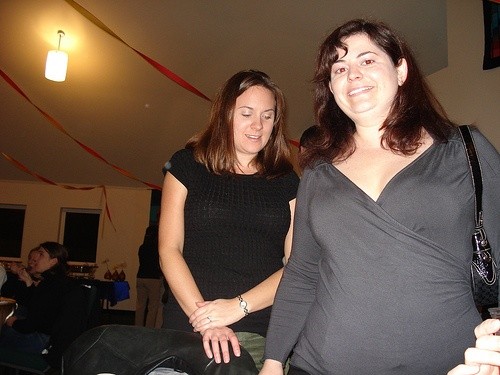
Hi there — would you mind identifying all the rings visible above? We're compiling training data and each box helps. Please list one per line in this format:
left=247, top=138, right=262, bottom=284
left=206, top=317, right=210, bottom=323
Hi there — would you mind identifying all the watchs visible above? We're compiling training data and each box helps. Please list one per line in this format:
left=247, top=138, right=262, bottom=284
left=235, top=295, right=249, bottom=315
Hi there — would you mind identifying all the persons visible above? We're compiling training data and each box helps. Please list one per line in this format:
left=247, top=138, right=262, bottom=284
left=259, top=19, right=499, bottom=375
left=133, top=225, right=163, bottom=330
left=158, top=69, right=301, bottom=374
left=0, top=239, right=82, bottom=375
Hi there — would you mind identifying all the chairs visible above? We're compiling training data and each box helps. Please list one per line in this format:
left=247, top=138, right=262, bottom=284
left=0, top=307, right=73, bottom=375
left=61, top=324, right=259, bottom=375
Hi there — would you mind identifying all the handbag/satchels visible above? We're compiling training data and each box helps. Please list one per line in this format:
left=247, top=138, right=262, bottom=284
left=458, top=125, right=500, bottom=319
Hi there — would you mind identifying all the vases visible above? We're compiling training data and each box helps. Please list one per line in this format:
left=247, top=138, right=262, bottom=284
left=118, top=271, right=126, bottom=281
left=112, top=271, right=119, bottom=281
left=104, top=271, right=112, bottom=280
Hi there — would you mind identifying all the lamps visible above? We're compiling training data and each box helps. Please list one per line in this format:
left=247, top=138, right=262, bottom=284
left=44, top=32, right=68, bottom=82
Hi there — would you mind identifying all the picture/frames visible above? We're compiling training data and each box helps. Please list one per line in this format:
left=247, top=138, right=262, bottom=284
left=482, top=0, right=500, bottom=70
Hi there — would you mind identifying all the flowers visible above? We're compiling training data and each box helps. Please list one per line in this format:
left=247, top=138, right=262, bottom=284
left=120, top=262, right=127, bottom=269
left=102, top=259, right=110, bottom=264
left=112, top=264, right=119, bottom=270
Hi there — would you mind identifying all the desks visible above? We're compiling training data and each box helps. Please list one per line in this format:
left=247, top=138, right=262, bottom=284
left=93, top=281, right=128, bottom=312
